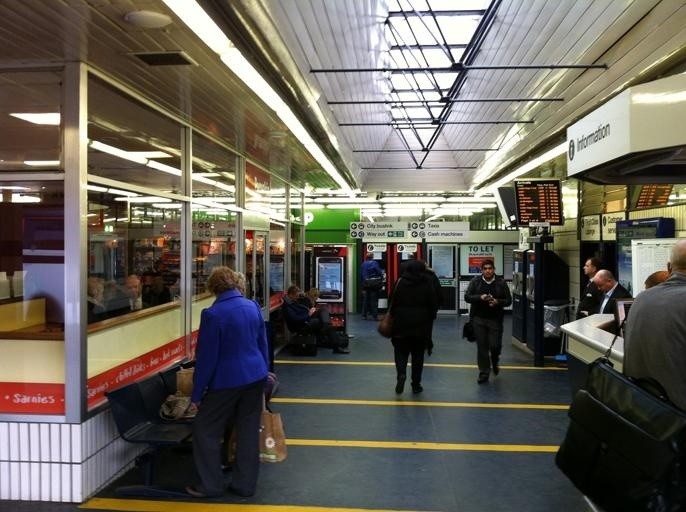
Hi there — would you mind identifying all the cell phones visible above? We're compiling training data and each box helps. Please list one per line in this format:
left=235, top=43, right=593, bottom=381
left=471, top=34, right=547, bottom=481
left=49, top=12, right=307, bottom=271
left=318, top=258, right=342, bottom=299
left=267, top=258, right=284, bottom=292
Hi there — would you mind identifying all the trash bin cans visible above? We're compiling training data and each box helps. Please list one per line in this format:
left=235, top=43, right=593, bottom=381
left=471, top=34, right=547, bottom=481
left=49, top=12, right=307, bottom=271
left=542, top=300, right=571, bottom=338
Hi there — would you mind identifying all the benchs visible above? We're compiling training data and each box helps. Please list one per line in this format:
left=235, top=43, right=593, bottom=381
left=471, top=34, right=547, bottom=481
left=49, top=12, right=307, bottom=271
left=104, top=356, right=211, bottom=497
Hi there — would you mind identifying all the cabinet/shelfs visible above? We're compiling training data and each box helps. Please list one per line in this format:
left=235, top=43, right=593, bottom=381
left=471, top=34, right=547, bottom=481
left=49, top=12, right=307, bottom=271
left=127, top=239, right=225, bottom=277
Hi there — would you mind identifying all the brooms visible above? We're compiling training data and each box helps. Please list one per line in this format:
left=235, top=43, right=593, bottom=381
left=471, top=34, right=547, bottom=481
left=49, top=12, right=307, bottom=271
left=553, top=311, right=568, bottom=362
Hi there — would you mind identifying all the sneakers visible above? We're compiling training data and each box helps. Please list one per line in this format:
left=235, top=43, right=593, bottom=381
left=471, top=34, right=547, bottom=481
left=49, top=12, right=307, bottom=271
left=477, top=369, right=490, bottom=384
left=395, top=377, right=406, bottom=394
left=492, top=361, right=499, bottom=376
left=411, top=382, right=423, bottom=394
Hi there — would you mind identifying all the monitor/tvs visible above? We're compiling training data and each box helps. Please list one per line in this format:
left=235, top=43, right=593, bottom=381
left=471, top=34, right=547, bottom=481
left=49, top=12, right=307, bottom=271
left=493, top=185, right=517, bottom=227
left=613, top=298, right=634, bottom=338
left=514, top=178, right=562, bottom=226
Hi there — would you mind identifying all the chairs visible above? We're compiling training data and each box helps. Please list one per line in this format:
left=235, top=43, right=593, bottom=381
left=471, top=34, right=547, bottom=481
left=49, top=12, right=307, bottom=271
left=266, top=309, right=318, bottom=356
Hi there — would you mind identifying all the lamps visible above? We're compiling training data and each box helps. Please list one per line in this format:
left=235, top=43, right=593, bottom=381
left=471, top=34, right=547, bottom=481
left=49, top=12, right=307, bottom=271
left=124, top=9, right=174, bottom=31
left=85, top=1, right=568, bottom=221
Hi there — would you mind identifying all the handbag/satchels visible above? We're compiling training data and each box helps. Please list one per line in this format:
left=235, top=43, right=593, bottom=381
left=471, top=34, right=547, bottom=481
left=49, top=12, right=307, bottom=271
left=377, top=314, right=392, bottom=338
left=228, top=410, right=288, bottom=464
left=159, top=394, right=197, bottom=420
left=553, top=356, right=682, bottom=511
left=462, top=321, right=474, bottom=343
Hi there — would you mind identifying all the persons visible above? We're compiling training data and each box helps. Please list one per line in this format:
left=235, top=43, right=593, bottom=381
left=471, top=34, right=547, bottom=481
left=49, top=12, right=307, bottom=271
left=576, top=257, right=604, bottom=320
left=183, top=267, right=269, bottom=497
left=464, top=259, right=512, bottom=384
left=388, top=259, right=442, bottom=394
left=593, top=269, right=633, bottom=328
left=359, top=252, right=383, bottom=322
left=623, top=239, right=686, bottom=413
left=89, top=260, right=172, bottom=323
left=281, top=286, right=350, bottom=354
left=644, top=271, right=672, bottom=289
